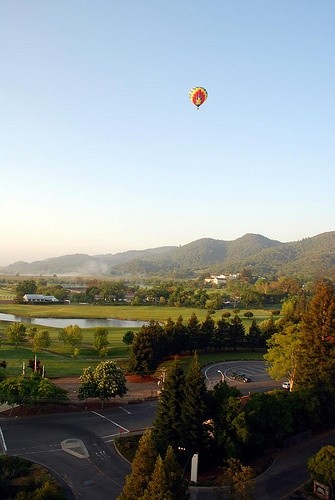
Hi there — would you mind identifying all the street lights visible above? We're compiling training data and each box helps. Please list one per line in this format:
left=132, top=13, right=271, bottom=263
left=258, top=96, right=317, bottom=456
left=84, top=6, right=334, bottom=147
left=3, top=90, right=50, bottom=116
left=218, top=369, right=224, bottom=383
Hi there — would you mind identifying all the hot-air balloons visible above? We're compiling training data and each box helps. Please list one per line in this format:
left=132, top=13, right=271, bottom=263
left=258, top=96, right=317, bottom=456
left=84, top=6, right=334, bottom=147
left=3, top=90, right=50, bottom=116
left=188, top=87, right=208, bottom=110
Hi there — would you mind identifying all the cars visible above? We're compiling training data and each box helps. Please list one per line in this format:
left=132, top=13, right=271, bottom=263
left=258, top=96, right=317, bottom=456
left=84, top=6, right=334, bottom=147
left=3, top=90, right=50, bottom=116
left=282, top=382, right=290, bottom=389
left=231, top=373, right=249, bottom=383
